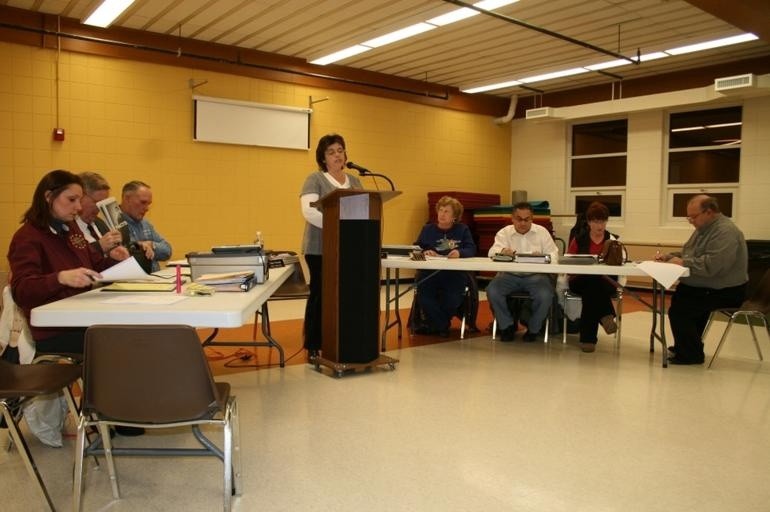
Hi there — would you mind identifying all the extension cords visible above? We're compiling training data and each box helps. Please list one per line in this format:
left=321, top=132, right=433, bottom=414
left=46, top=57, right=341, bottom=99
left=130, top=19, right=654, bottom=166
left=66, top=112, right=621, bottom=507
left=233, top=346, right=258, bottom=364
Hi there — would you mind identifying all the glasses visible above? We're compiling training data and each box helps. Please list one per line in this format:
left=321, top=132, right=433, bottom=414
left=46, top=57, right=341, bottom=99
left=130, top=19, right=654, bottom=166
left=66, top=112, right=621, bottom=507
left=687, top=207, right=707, bottom=221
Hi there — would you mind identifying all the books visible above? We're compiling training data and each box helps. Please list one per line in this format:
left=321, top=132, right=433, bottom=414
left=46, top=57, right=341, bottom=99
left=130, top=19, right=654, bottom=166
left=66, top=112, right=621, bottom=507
left=557, top=254, right=600, bottom=265
left=425, top=254, right=449, bottom=262
left=492, top=254, right=513, bottom=262
left=99, top=259, right=191, bottom=291
left=513, top=253, right=551, bottom=265
left=197, top=272, right=254, bottom=284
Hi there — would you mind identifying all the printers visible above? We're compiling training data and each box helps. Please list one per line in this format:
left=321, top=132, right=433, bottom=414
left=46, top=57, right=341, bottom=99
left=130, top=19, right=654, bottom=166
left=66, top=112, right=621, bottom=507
left=185, top=244, right=270, bottom=285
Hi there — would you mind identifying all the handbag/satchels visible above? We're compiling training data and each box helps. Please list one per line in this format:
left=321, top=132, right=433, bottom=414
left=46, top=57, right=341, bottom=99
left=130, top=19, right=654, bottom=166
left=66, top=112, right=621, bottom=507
left=597, top=239, right=629, bottom=266
left=126, top=241, right=152, bottom=274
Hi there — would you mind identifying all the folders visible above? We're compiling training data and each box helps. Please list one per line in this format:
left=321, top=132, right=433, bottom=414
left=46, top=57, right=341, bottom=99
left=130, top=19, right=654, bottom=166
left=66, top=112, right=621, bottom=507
left=99, top=275, right=186, bottom=292
left=196, top=270, right=257, bottom=292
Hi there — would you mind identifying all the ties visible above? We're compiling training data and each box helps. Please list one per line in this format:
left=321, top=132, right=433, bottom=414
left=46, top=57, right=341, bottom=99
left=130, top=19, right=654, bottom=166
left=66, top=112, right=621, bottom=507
left=87, top=225, right=102, bottom=240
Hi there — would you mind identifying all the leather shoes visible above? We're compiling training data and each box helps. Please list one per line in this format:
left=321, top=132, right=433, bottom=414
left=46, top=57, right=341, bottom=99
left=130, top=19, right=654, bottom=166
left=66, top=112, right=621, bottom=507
left=502, top=328, right=513, bottom=341
left=668, top=357, right=704, bottom=364
left=307, top=349, right=320, bottom=366
left=522, top=329, right=536, bottom=342
left=668, top=346, right=677, bottom=353
left=114, top=426, right=144, bottom=437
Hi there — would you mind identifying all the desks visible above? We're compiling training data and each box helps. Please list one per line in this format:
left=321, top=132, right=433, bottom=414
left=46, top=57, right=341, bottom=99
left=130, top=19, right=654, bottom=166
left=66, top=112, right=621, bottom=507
left=30, top=264, right=295, bottom=496
left=381, top=256, right=690, bottom=368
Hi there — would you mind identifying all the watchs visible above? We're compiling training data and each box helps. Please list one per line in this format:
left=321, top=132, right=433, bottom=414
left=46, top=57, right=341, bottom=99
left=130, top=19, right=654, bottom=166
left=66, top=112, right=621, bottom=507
left=152, top=241, right=157, bottom=252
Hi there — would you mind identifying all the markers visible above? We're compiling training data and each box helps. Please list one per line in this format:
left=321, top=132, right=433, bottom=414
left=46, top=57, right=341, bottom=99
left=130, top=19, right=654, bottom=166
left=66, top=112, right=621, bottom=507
left=176, top=264, right=181, bottom=293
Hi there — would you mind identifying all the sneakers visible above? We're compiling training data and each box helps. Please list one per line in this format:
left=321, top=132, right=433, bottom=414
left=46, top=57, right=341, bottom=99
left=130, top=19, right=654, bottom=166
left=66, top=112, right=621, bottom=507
left=582, top=343, right=594, bottom=352
left=602, top=317, right=617, bottom=334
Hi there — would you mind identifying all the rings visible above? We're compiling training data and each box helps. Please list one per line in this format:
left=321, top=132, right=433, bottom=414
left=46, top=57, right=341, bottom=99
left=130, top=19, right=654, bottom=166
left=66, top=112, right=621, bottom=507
left=85, top=269, right=91, bottom=275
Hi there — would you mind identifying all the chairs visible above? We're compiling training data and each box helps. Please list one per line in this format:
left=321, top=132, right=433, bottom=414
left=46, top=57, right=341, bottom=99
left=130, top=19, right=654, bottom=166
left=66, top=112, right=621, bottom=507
left=72, top=324, right=242, bottom=512
left=7, top=354, right=100, bottom=469
left=492, top=238, right=565, bottom=343
left=0, top=359, right=100, bottom=512
left=562, top=242, right=628, bottom=347
left=410, top=280, right=470, bottom=339
left=702, top=268, right=770, bottom=368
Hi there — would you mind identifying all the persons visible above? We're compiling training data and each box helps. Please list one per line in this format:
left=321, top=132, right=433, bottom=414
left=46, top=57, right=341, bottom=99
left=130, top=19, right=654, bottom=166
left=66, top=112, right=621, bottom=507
left=299, top=133, right=364, bottom=365
left=413, top=197, right=478, bottom=339
left=653, top=195, right=749, bottom=366
left=485, top=203, right=559, bottom=344
left=7, top=170, right=145, bottom=437
left=566, top=203, right=619, bottom=354
left=73, top=171, right=155, bottom=273
left=119, top=181, right=172, bottom=274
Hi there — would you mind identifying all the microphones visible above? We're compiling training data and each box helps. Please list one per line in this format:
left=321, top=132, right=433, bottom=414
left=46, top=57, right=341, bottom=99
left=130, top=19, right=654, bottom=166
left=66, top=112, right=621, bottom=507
left=346, top=160, right=370, bottom=177
